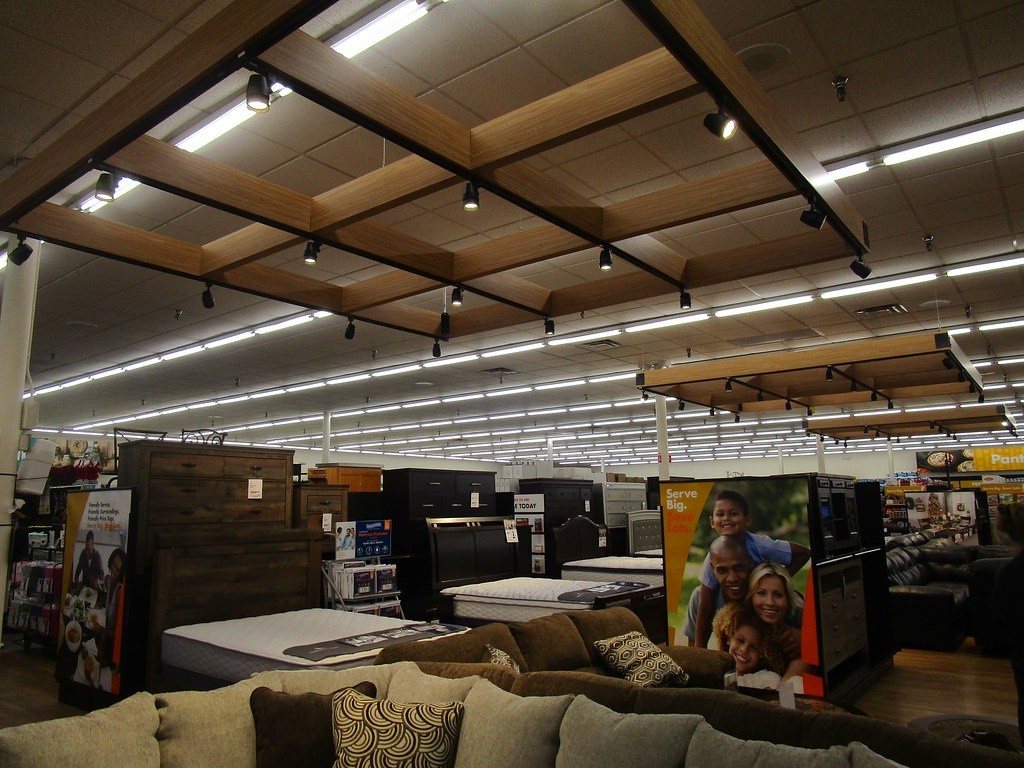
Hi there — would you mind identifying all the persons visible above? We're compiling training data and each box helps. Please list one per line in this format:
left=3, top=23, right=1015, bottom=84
left=74, top=530, right=124, bottom=640
left=997, top=505, right=1024, bottom=748
left=682, top=490, right=810, bottom=690
left=336, top=527, right=356, bottom=552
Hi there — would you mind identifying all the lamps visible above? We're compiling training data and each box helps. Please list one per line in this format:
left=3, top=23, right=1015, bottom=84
left=800, top=204, right=827, bottom=230
left=433, top=338, right=441, bottom=357
left=679, top=289, right=691, bottom=309
left=543, top=317, right=555, bottom=335
left=703, top=107, right=736, bottom=140
left=462, top=183, right=480, bottom=211
left=0, top=0, right=449, bottom=264
left=640, top=306, right=1024, bottom=449
left=201, top=283, right=215, bottom=308
left=452, top=287, right=465, bottom=305
left=831, top=77, right=933, bottom=251
left=850, top=249, right=872, bottom=280
left=598, top=249, right=612, bottom=270
left=344, top=316, right=355, bottom=340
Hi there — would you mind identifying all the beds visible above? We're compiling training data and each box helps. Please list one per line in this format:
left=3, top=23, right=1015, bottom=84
left=147, top=529, right=473, bottom=693
left=426, top=518, right=654, bottom=628
left=562, top=510, right=662, bottom=587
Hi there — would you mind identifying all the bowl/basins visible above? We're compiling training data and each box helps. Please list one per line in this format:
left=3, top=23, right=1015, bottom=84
left=65, top=620, right=82, bottom=652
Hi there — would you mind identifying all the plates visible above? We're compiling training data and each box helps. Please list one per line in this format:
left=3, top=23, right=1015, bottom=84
left=89, top=611, right=103, bottom=630
left=64, top=592, right=74, bottom=608
left=83, top=588, right=95, bottom=602
left=78, top=652, right=98, bottom=680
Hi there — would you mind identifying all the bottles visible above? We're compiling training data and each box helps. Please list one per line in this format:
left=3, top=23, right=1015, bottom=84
left=62, top=455, right=71, bottom=467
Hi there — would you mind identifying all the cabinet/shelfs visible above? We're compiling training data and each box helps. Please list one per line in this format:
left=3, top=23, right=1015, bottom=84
left=886, top=504, right=906, bottom=513
left=116, top=440, right=648, bottom=698
left=904, top=490, right=992, bottom=546
left=659, top=473, right=893, bottom=703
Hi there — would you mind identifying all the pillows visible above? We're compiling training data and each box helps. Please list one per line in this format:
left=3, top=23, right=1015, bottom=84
left=847, top=741, right=909, bottom=768
left=555, top=694, right=705, bottom=768
left=332, top=688, right=465, bottom=768
left=684, top=721, right=850, bottom=768
left=249, top=682, right=377, bottom=768
left=482, top=644, right=520, bottom=674
left=454, top=679, right=575, bottom=768
left=594, top=630, right=689, bottom=688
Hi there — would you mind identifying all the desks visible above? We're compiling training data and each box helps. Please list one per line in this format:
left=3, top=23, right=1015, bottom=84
left=908, top=714, right=1024, bottom=753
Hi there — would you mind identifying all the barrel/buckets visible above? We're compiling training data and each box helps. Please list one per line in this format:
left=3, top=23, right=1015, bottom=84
left=16, top=459, right=51, bottom=497
left=30, top=439, right=57, bottom=466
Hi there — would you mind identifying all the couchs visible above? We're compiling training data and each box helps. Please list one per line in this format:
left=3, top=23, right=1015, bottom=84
left=0, top=606, right=1024, bottom=768
left=886, top=533, right=1014, bottom=652
